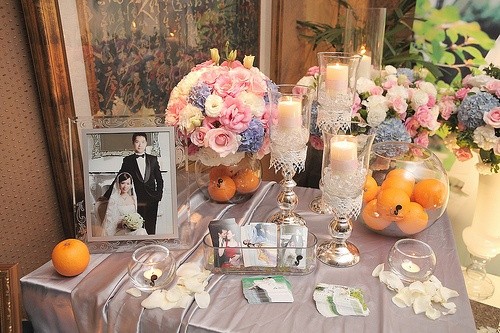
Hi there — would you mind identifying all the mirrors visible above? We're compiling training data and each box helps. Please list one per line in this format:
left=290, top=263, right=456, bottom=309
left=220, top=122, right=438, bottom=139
left=91, top=133, right=162, bottom=160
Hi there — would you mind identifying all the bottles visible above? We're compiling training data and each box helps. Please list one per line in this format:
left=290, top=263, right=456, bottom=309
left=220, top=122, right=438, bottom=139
left=359, top=140, right=450, bottom=237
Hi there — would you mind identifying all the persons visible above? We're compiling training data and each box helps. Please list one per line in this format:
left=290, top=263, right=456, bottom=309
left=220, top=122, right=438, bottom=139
left=96, top=131, right=165, bottom=237
left=214, top=224, right=305, bottom=268
left=254, top=277, right=277, bottom=290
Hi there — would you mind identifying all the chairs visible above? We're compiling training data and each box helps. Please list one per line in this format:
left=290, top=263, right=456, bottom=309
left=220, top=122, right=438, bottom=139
left=94, top=199, right=109, bottom=226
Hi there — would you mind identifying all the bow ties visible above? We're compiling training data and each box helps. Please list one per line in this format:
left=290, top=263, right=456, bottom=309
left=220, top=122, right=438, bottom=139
left=136, top=154, right=144, bottom=158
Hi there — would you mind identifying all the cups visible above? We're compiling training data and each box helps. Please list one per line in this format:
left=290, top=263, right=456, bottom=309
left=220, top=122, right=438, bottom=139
left=387, top=238, right=438, bottom=284
left=128, top=244, right=177, bottom=292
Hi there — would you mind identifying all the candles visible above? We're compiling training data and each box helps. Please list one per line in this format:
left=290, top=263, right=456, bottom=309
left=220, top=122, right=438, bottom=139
left=327, top=63, right=349, bottom=90
left=331, top=139, right=357, bottom=160
left=352, top=49, right=372, bottom=75
left=278, top=97, right=301, bottom=128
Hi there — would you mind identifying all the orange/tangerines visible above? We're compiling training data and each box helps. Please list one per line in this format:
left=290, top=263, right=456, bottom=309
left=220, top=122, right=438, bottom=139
left=209, top=166, right=259, bottom=201
left=363, top=169, right=446, bottom=234
left=52, top=239, right=91, bottom=277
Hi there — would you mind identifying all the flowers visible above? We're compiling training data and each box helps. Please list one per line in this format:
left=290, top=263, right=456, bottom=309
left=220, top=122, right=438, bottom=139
left=292, top=62, right=500, bottom=174
left=166, top=40, right=281, bottom=166
left=120, top=213, right=144, bottom=230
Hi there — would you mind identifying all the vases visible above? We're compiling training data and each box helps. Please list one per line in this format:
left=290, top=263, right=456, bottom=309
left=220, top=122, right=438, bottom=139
left=194, top=147, right=265, bottom=203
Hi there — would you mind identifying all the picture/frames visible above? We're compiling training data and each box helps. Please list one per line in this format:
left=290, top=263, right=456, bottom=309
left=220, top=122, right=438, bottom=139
left=21, top=0, right=285, bottom=239
left=67, top=113, right=192, bottom=256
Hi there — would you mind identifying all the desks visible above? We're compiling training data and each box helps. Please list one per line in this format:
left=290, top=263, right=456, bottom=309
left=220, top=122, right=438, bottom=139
left=20, top=174, right=478, bottom=333
